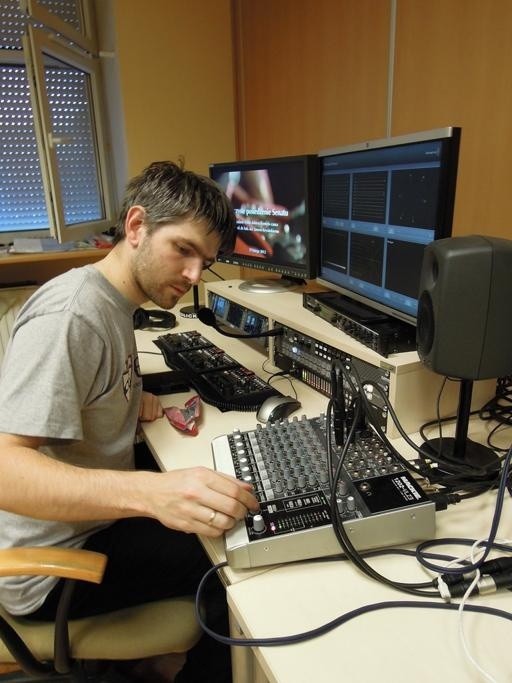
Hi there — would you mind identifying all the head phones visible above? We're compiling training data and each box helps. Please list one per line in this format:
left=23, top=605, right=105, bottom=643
left=131, top=307, right=178, bottom=330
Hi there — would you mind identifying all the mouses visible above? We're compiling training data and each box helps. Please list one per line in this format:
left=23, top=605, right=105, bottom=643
left=255, top=391, right=300, bottom=425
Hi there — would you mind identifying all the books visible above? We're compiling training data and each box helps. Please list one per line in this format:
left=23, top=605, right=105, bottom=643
left=12, top=237, right=67, bottom=252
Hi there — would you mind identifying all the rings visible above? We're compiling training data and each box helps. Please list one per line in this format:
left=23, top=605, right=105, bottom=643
left=207, top=507, right=216, bottom=523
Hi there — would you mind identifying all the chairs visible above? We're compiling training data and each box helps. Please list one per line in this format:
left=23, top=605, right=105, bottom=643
left=0, top=546, right=207, bottom=683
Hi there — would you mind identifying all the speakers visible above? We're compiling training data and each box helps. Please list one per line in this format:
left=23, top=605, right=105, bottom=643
left=413, top=232, right=512, bottom=380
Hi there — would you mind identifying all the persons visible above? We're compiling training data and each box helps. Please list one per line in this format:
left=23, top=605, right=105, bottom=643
left=0, top=154, right=263, bottom=683
left=218, top=168, right=277, bottom=257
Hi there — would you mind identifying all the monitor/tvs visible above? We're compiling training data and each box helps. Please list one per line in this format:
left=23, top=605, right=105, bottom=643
left=207, top=156, right=322, bottom=293
left=312, top=126, right=463, bottom=327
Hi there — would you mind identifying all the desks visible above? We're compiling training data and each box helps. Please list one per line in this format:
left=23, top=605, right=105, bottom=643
left=129, top=275, right=512, bottom=683
